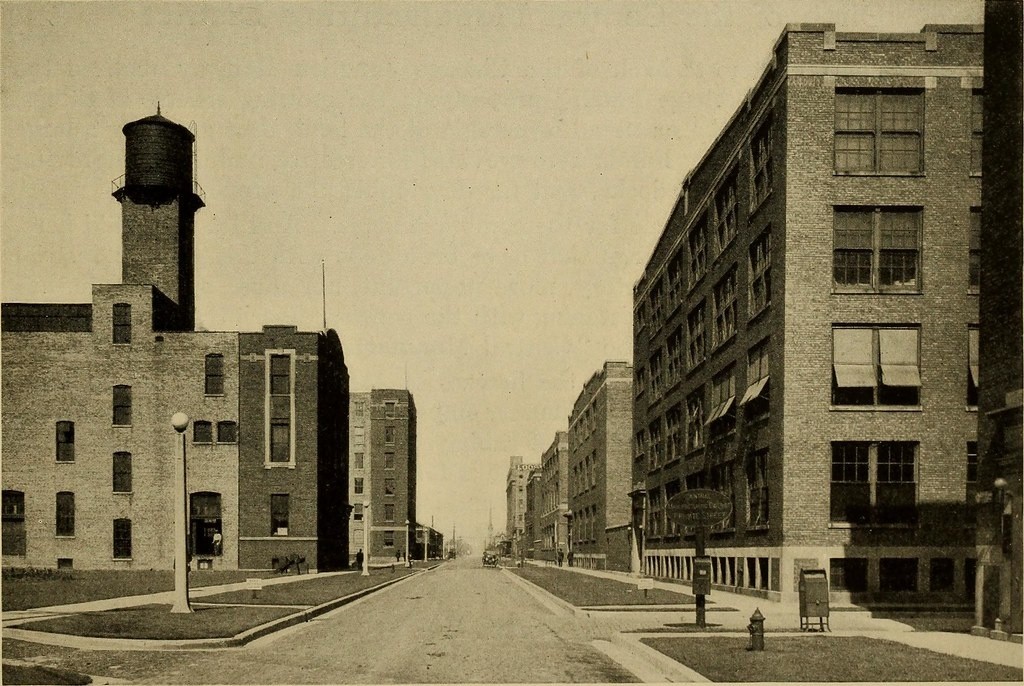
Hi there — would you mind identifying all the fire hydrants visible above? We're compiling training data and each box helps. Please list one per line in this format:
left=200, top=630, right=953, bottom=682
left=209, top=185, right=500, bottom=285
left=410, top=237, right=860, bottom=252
left=747, top=607, right=766, bottom=651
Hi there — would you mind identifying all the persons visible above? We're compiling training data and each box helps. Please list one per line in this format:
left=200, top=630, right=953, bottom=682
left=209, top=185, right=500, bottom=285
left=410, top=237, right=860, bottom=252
left=212, top=530, right=222, bottom=556
left=356, top=549, right=364, bottom=570
left=395, top=550, right=406, bottom=562
left=567, top=549, right=574, bottom=567
left=558, top=549, right=564, bottom=567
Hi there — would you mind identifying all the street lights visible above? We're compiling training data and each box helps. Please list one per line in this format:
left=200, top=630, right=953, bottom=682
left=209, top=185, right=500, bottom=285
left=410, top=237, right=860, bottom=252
left=442, top=537, right=446, bottom=559
left=169, top=411, right=194, bottom=614
left=361, top=500, right=371, bottom=576
left=424, top=528, right=428, bottom=562
left=405, top=518, right=411, bottom=568
